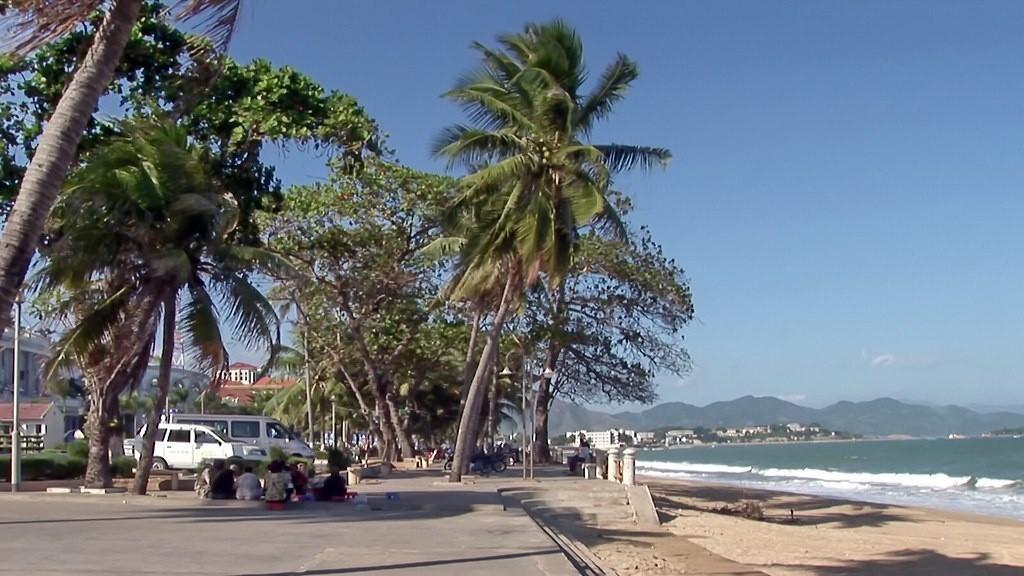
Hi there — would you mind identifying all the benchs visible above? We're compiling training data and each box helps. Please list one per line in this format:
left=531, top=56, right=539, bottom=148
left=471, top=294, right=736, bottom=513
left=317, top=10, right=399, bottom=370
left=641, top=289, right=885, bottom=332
left=131, top=468, right=183, bottom=491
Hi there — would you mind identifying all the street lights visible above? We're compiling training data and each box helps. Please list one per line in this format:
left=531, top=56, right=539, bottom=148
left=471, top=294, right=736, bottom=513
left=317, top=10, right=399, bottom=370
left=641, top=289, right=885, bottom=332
left=10, top=291, right=28, bottom=495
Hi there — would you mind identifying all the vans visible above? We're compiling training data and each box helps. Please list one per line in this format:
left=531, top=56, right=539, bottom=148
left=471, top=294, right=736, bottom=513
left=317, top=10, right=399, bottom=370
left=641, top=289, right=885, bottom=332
left=168, top=414, right=316, bottom=467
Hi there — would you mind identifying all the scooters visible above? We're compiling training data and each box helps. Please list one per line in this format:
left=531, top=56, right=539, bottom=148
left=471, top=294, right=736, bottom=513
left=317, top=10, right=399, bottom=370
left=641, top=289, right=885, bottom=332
left=444, top=447, right=520, bottom=473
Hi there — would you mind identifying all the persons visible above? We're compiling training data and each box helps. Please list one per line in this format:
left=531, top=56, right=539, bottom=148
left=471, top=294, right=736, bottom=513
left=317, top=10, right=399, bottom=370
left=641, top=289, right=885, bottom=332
left=568, top=441, right=590, bottom=476
left=200, top=461, right=346, bottom=504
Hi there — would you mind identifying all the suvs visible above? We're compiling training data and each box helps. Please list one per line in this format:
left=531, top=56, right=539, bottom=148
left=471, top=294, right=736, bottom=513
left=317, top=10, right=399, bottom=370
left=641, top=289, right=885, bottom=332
left=135, top=423, right=268, bottom=472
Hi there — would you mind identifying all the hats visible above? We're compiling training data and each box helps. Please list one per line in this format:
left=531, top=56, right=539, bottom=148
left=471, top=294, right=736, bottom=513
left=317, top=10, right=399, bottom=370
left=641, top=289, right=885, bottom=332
left=230, top=464, right=237, bottom=471
